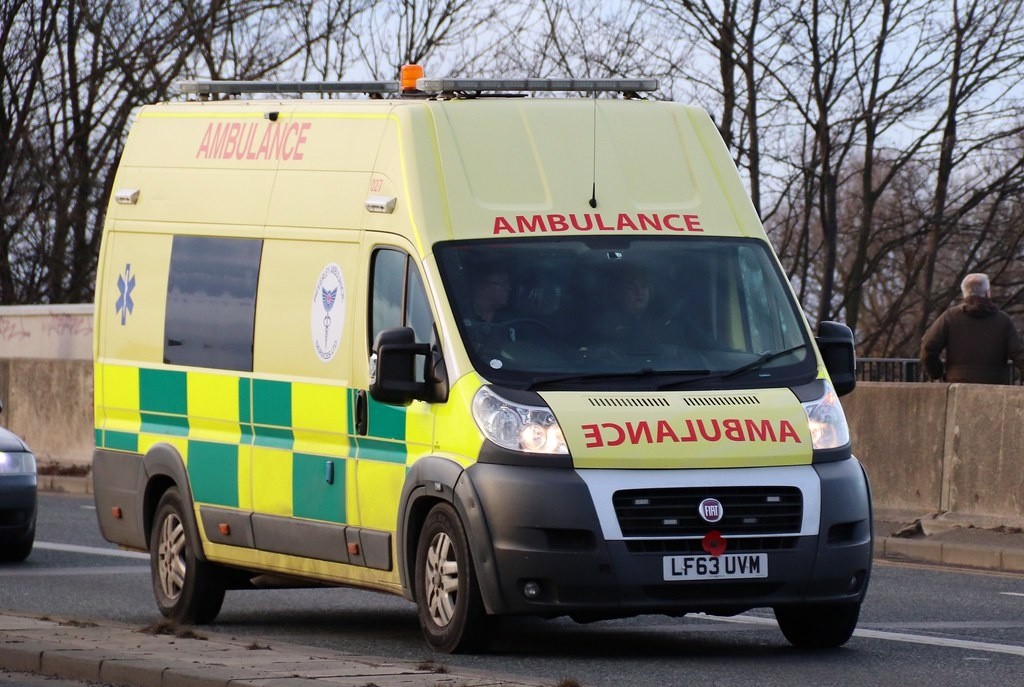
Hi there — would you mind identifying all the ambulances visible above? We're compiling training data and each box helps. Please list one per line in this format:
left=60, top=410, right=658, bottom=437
left=90, top=58, right=872, bottom=649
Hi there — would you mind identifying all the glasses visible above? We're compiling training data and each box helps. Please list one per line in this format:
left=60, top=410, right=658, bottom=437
left=485, top=279, right=513, bottom=288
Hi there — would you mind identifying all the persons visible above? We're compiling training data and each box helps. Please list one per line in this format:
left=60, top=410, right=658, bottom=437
left=594, top=273, right=684, bottom=346
left=428, top=258, right=543, bottom=380
left=921, top=274, right=1024, bottom=386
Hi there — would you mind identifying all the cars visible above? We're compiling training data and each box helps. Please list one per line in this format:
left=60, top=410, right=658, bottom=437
left=4, top=428, right=40, bottom=563
